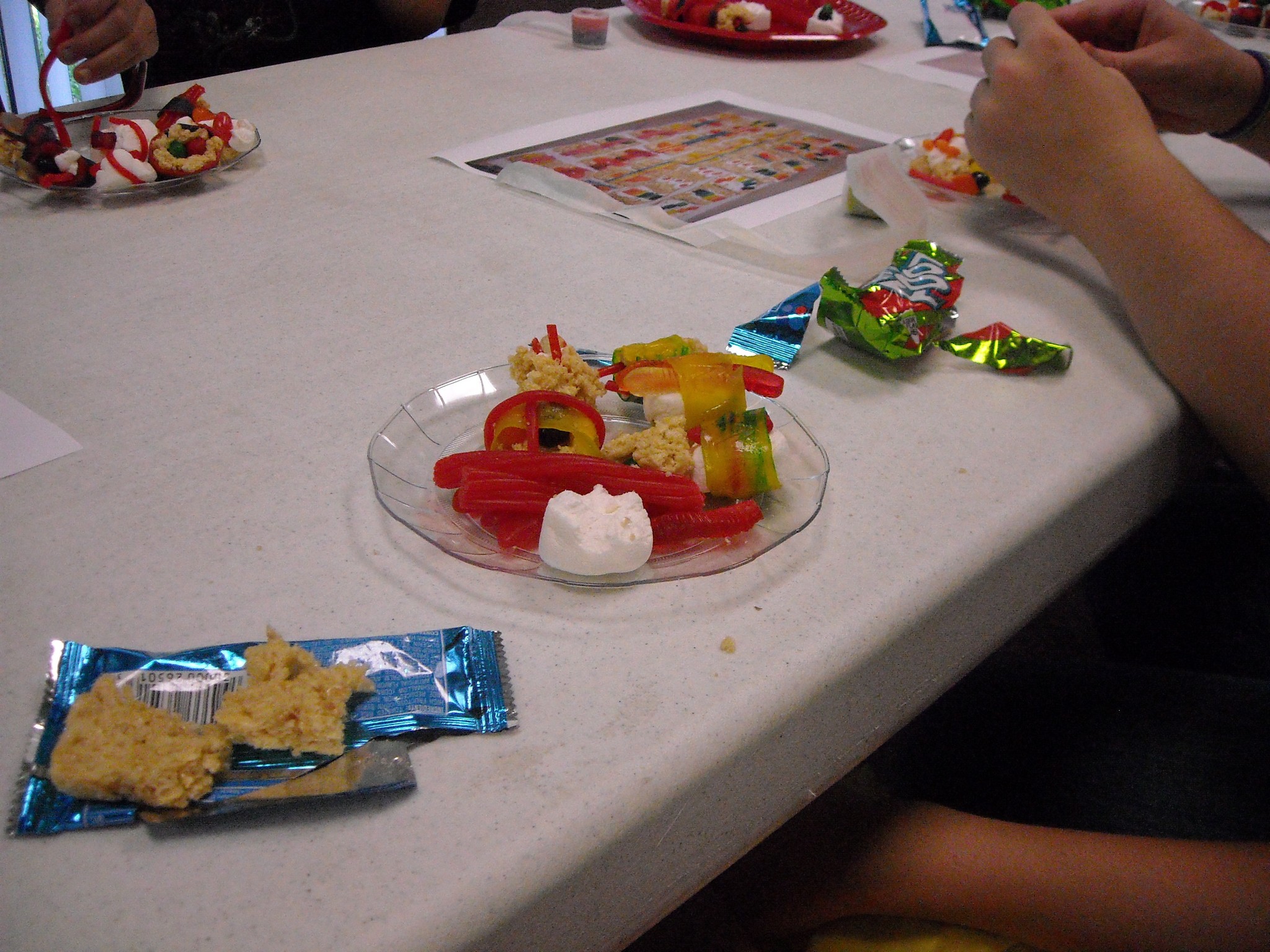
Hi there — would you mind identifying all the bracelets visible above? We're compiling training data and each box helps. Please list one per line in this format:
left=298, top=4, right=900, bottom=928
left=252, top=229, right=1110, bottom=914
left=1209, top=49, right=1270, bottom=138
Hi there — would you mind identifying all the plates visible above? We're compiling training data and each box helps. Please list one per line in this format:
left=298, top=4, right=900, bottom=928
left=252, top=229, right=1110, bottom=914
left=1176, top=0, right=1270, bottom=39
left=622, top=0, right=888, bottom=50
left=0, top=108, right=261, bottom=198
left=892, top=132, right=1064, bottom=236
left=366, top=363, right=830, bottom=589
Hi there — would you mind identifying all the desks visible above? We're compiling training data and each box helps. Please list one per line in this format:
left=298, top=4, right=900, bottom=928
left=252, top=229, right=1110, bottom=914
left=0, top=2, right=1270, bottom=952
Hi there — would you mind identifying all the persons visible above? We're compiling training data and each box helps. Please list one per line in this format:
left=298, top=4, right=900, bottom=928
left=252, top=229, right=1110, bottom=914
left=735, top=782, right=1270, bottom=952
left=964, top=0, right=1270, bottom=504
left=0, top=0, right=477, bottom=127
